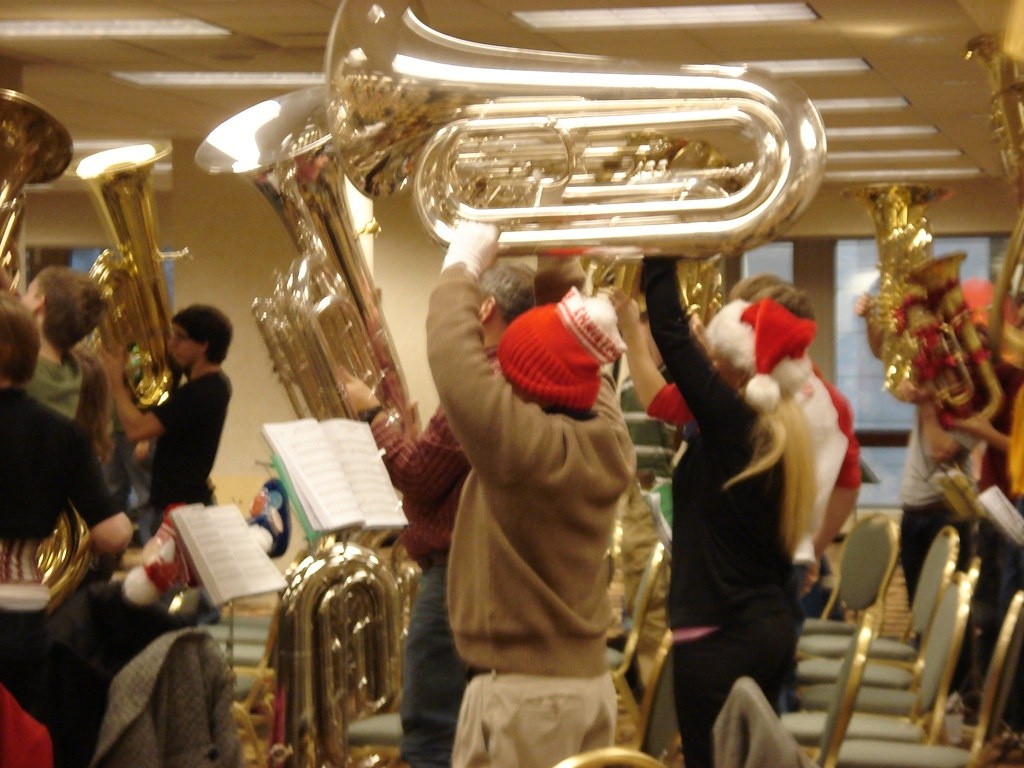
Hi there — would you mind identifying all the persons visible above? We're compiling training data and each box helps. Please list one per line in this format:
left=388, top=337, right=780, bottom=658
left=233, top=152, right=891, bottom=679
left=0, top=267, right=234, bottom=768
left=898, top=279, right=1024, bottom=735
left=608, top=255, right=863, bottom=767
left=340, top=218, right=636, bottom=768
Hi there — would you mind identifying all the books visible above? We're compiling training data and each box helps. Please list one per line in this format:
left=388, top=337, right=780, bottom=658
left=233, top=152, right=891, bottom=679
left=169, top=501, right=289, bottom=606
left=259, top=418, right=410, bottom=541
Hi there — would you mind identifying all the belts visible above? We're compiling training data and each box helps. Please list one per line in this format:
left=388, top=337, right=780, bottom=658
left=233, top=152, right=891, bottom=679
left=417, top=551, right=448, bottom=569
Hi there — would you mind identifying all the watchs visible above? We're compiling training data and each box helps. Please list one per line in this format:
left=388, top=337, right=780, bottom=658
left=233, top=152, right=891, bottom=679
left=357, top=406, right=382, bottom=424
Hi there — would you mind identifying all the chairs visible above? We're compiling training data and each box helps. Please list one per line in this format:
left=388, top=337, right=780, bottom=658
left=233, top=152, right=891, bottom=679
left=198, top=509, right=1024, bottom=768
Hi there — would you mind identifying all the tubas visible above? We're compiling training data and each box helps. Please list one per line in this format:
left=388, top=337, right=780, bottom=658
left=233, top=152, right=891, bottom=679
left=194, top=87, right=418, bottom=447
left=60, top=136, right=182, bottom=410
left=0, top=83, right=74, bottom=293
left=843, top=179, right=957, bottom=358
left=324, top=0, right=827, bottom=262
left=893, top=249, right=1003, bottom=428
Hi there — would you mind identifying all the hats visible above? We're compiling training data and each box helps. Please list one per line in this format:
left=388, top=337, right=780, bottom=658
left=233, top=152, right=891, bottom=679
left=497, top=286, right=627, bottom=411
left=705, top=297, right=819, bottom=414
